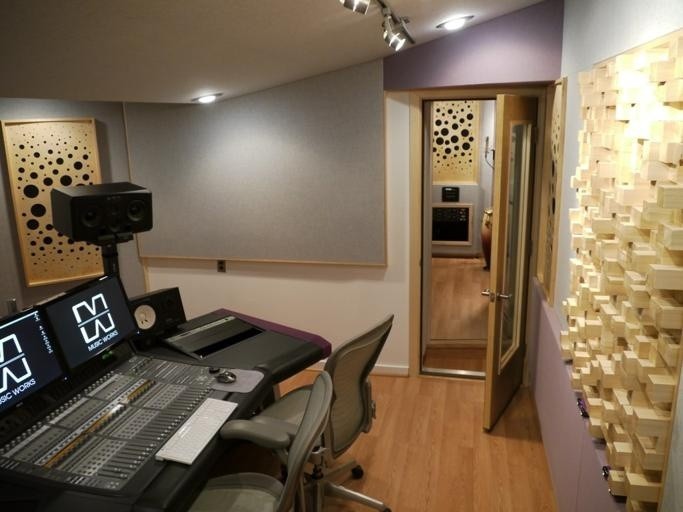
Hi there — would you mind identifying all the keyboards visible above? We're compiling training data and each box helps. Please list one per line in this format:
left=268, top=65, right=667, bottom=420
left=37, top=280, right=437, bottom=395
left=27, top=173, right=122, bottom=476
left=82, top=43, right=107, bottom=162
left=155, top=398, right=238, bottom=465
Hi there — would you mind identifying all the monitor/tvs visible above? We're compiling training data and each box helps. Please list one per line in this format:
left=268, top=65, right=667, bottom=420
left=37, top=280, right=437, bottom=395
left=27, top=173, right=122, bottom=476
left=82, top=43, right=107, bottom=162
left=0, top=308, right=67, bottom=420
left=33, top=272, right=139, bottom=377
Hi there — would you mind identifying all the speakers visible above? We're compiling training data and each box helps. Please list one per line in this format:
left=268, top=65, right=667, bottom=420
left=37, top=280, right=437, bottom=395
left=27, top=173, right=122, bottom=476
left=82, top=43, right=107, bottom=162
left=129, top=287, right=186, bottom=351
left=50, top=182, right=153, bottom=242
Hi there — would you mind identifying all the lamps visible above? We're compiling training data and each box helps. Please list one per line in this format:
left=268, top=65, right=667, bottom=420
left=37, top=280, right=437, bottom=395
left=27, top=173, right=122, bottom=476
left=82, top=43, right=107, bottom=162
left=338, top=0, right=393, bottom=16
left=381, top=15, right=416, bottom=51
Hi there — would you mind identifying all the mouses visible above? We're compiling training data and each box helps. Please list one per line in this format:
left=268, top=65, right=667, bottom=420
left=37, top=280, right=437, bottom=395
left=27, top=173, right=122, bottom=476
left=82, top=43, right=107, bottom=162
left=215, top=371, right=236, bottom=383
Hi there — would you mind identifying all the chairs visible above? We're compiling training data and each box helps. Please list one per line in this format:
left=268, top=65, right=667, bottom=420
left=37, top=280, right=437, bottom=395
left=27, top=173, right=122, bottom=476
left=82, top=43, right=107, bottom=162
left=184, top=368, right=336, bottom=512
left=249, top=305, right=395, bottom=511
